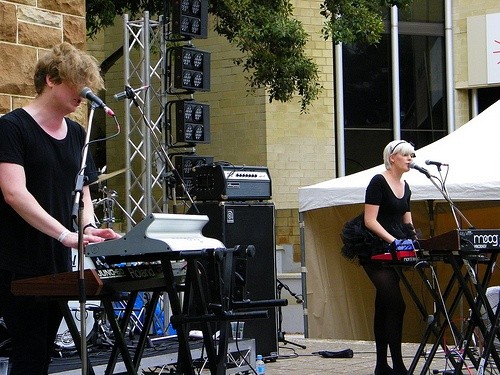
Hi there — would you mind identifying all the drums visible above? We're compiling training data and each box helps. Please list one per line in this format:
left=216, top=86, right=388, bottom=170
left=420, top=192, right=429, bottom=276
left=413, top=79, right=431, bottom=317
left=53, top=246, right=106, bottom=352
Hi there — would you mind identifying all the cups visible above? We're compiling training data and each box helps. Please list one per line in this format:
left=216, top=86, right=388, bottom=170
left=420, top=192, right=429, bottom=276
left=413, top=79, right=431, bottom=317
left=0, top=357, right=9, bottom=375
left=230, top=321, right=245, bottom=340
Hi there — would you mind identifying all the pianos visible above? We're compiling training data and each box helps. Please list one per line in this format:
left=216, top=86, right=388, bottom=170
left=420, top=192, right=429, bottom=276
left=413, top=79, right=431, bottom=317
left=367, top=227, right=500, bottom=375
left=9, top=214, right=229, bottom=375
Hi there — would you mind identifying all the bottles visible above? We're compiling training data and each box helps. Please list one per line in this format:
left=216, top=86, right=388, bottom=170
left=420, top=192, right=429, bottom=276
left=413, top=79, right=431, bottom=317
left=256, top=355, right=265, bottom=375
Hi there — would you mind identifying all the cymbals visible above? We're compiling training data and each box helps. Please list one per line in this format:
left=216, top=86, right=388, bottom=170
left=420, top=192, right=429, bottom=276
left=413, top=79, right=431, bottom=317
left=83, top=165, right=132, bottom=188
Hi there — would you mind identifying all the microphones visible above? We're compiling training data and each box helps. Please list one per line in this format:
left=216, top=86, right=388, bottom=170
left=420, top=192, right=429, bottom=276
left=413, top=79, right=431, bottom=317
left=409, top=161, right=429, bottom=174
left=425, top=160, right=442, bottom=166
left=113, top=85, right=150, bottom=102
left=296, top=299, right=305, bottom=304
left=80, top=87, right=115, bottom=116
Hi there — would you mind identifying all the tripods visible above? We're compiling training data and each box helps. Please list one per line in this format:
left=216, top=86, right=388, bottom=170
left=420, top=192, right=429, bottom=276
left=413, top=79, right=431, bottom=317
left=70, top=308, right=141, bottom=356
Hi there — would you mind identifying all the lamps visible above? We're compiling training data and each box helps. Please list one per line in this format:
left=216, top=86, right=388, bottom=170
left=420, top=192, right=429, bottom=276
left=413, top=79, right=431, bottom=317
left=165, top=99, right=210, bottom=148
left=167, top=47, right=210, bottom=94
left=166, top=153, right=213, bottom=200
left=165, top=0, right=207, bottom=42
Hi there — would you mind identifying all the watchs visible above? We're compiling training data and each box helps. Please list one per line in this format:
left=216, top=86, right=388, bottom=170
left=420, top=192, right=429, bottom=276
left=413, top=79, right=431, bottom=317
left=83, top=222, right=98, bottom=234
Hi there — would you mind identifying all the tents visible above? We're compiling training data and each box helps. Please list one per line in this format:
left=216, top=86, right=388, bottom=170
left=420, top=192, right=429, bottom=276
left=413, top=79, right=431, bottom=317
left=297, top=97, right=500, bottom=341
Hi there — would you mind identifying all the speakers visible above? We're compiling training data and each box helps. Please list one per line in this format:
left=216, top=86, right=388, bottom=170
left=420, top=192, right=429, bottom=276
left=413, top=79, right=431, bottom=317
left=185, top=201, right=278, bottom=361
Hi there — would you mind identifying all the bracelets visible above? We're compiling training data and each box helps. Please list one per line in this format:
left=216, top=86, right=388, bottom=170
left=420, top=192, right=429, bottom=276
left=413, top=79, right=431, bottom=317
left=57, top=229, right=69, bottom=242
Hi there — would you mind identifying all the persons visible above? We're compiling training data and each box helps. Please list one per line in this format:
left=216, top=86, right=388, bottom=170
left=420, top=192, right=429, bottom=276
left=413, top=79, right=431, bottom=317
left=475, top=286, right=500, bottom=358
left=0, top=40, right=121, bottom=375
left=361, top=140, right=418, bottom=375
